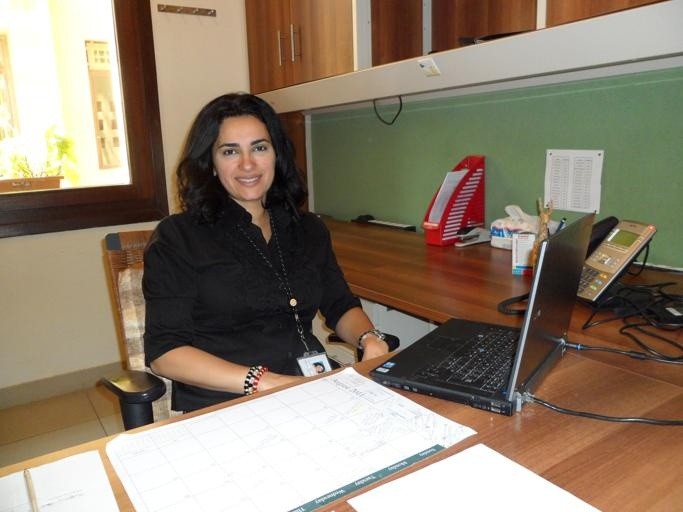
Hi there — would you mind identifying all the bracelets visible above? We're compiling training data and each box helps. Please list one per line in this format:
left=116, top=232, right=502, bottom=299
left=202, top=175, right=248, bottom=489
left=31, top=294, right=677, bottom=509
left=358, top=328, right=387, bottom=346
left=244, top=364, right=269, bottom=396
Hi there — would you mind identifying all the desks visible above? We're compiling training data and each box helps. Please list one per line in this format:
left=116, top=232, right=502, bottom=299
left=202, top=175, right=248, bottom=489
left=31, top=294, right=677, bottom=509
left=0, top=317, right=680, bottom=512
left=304, top=203, right=680, bottom=379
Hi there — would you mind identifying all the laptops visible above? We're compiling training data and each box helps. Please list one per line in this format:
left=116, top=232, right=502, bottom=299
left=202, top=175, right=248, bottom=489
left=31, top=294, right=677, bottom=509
left=368, top=210, right=597, bottom=416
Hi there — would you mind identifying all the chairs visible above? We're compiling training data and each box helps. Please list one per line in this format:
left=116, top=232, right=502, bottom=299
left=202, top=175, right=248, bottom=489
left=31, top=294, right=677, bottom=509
left=94, top=225, right=184, bottom=433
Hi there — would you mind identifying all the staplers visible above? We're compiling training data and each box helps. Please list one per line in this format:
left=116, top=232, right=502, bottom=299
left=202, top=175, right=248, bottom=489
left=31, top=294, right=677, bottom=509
left=454, top=227, right=492, bottom=247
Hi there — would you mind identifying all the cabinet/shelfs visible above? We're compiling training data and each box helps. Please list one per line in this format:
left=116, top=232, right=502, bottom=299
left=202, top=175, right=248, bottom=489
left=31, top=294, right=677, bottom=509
left=360, top=1, right=682, bottom=67
left=246, top=1, right=357, bottom=96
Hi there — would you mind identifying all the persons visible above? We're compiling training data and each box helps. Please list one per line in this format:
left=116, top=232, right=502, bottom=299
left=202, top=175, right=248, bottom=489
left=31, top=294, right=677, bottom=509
left=141, top=92, right=390, bottom=413
left=313, top=361, right=326, bottom=374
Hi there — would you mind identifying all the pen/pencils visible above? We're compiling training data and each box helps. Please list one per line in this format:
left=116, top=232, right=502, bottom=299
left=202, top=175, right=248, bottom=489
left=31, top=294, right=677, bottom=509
left=555, top=218, right=566, bottom=234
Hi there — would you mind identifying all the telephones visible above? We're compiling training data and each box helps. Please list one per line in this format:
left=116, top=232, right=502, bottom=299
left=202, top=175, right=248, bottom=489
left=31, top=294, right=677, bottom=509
left=577, top=216, right=657, bottom=307
